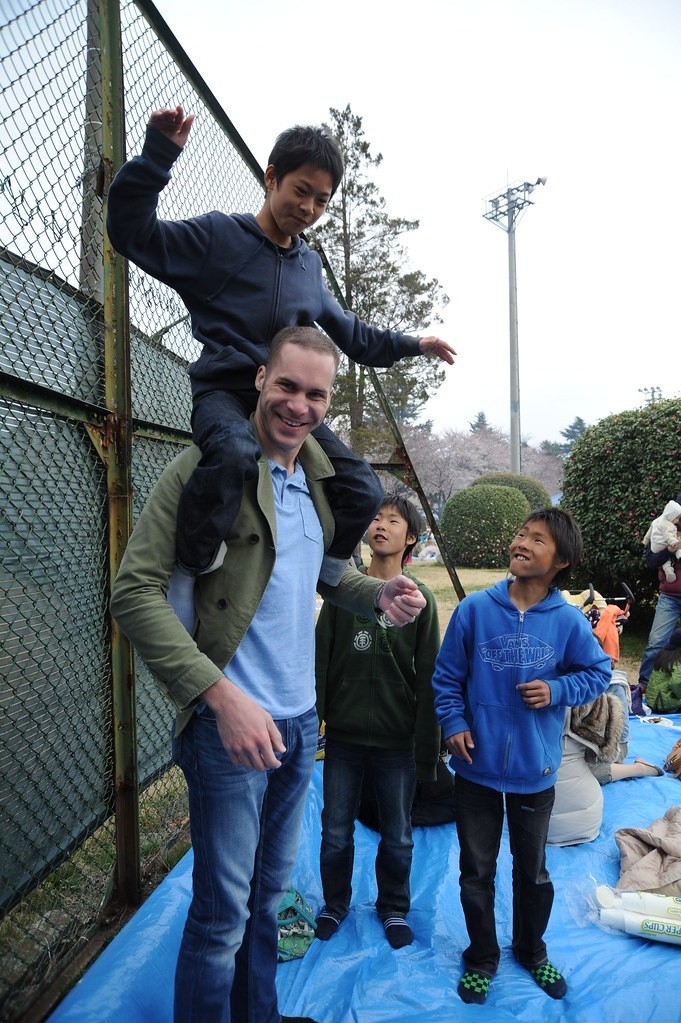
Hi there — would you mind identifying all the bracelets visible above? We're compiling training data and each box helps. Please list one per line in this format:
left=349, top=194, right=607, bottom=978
left=376, top=589, right=383, bottom=600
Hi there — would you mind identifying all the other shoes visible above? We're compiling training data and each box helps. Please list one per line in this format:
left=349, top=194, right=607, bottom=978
left=634, top=759, right=664, bottom=776
left=642, top=696, right=647, bottom=705
left=667, top=573, right=676, bottom=583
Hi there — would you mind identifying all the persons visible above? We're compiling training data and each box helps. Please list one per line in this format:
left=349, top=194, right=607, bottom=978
left=639, top=493, right=681, bottom=703
left=107, top=107, right=458, bottom=632
left=312, top=494, right=441, bottom=948
left=432, top=509, right=610, bottom=1005
left=547, top=635, right=665, bottom=847
left=109, top=325, right=428, bottom=1023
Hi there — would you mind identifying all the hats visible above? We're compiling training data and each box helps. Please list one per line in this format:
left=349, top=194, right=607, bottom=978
left=665, top=628, right=681, bottom=650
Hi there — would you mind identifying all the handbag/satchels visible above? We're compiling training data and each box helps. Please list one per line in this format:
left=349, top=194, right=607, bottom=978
left=276, top=884, right=318, bottom=963
left=663, top=739, right=681, bottom=780
